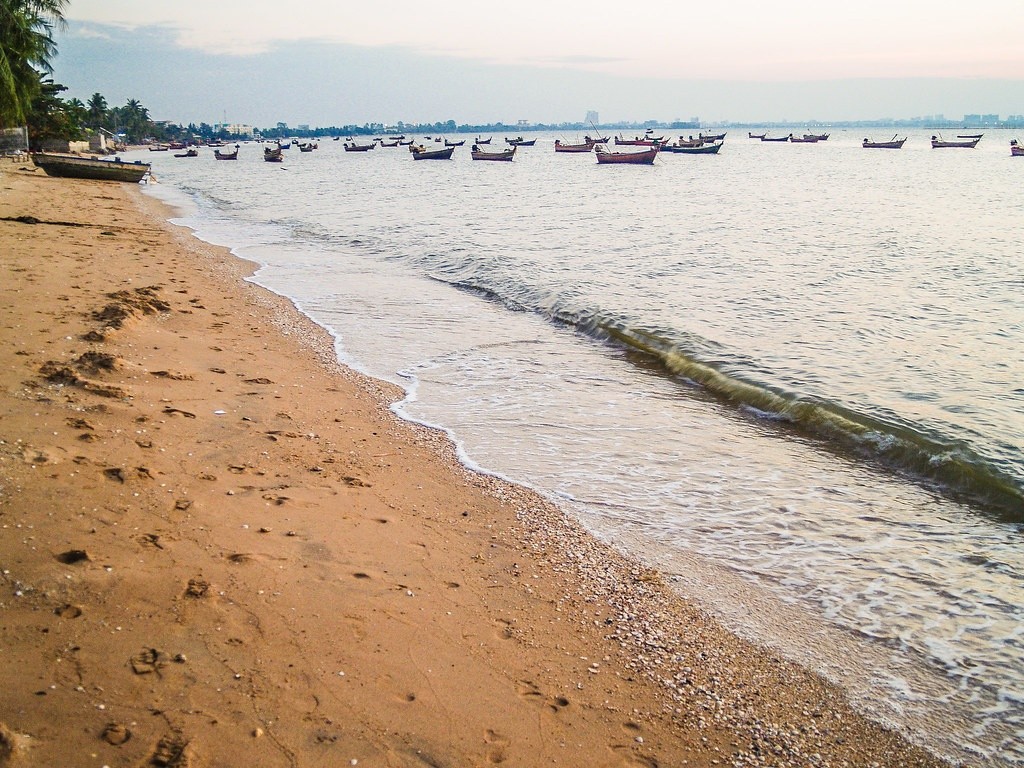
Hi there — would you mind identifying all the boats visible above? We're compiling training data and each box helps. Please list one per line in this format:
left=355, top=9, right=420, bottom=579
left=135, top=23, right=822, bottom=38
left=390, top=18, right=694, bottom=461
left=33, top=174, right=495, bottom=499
left=1010, top=138, right=1024, bottom=156
left=931, top=131, right=985, bottom=148
left=474, top=134, right=494, bottom=146
left=435, top=135, right=467, bottom=146
left=333, top=133, right=415, bottom=152
left=584, top=134, right=611, bottom=143
left=555, top=139, right=597, bottom=152
left=593, top=145, right=661, bottom=165
left=615, top=133, right=727, bottom=154
left=471, top=144, right=519, bottom=162
left=29, top=149, right=152, bottom=183
left=410, top=147, right=454, bottom=161
left=748, top=130, right=831, bottom=143
left=504, top=136, right=538, bottom=146
left=149, top=137, right=319, bottom=162
left=862, top=133, right=908, bottom=148
